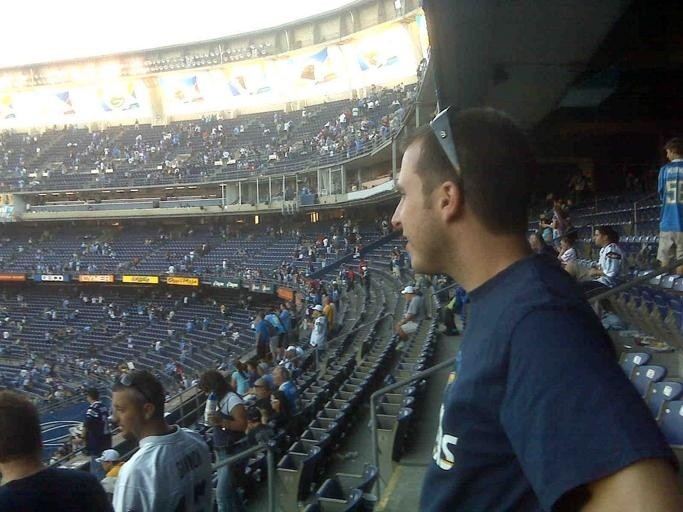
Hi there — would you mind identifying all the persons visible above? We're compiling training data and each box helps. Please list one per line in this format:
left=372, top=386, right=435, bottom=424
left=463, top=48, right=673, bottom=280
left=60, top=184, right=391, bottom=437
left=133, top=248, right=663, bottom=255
left=0, top=81, right=683, bottom=512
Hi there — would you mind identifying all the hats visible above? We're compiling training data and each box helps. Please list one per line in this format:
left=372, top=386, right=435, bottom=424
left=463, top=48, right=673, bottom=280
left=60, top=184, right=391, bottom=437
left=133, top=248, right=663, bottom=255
left=401, top=286, right=414, bottom=294
left=95, top=449, right=120, bottom=462
left=246, top=405, right=261, bottom=421
left=312, top=305, right=323, bottom=312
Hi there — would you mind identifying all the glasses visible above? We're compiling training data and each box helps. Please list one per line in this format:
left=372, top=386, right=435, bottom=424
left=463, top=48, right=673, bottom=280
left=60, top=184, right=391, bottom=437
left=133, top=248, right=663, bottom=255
left=429, top=104, right=461, bottom=179
left=119, top=373, right=151, bottom=402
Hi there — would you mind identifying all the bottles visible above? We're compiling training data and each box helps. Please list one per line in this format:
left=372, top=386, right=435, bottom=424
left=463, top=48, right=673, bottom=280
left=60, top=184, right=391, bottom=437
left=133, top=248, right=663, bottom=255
left=302, top=319, right=307, bottom=330
left=202, top=391, right=218, bottom=427
